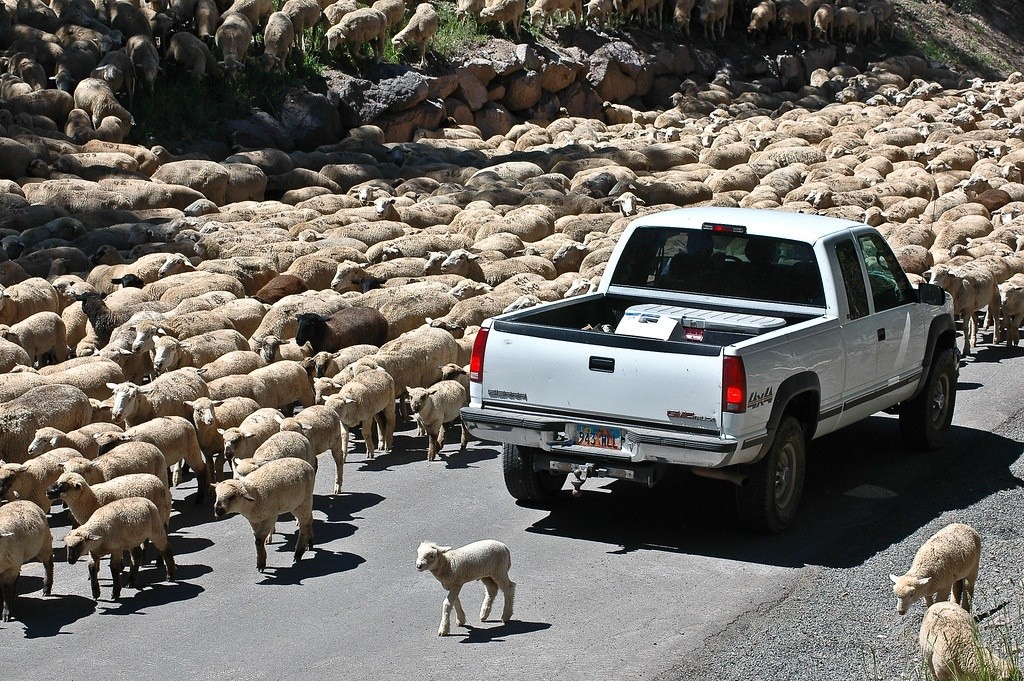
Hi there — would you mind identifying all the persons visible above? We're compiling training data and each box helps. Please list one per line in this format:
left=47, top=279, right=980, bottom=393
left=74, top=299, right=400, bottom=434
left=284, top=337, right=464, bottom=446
left=669, top=232, right=733, bottom=291
left=745, top=238, right=776, bottom=263
left=836, top=238, right=884, bottom=318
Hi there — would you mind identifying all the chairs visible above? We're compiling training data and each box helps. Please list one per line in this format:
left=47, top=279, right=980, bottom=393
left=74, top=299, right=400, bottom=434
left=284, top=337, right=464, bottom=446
left=654, top=275, right=684, bottom=290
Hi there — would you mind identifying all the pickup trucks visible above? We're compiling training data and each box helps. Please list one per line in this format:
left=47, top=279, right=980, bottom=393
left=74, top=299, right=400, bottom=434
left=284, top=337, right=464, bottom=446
left=461, top=207, right=961, bottom=536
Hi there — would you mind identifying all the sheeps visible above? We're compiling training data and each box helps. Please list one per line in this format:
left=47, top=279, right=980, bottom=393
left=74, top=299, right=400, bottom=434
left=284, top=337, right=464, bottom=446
left=56, top=441, right=169, bottom=488
left=415, top=540, right=516, bottom=636
left=45, top=472, right=167, bottom=530
left=209, top=459, right=315, bottom=572
left=27, top=422, right=124, bottom=462
left=0, top=500, right=54, bottom=623
left=56, top=496, right=176, bottom=602
left=0, top=447, right=82, bottom=514
left=234, top=431, right=313, bottom=480
left=888, top=523, right=981, bottom=620
left=919, top=602, right=1008, bottom=681
left=94, top=416, right=207, bottom=498
left=216, top=408, right=284, bottom=472
left=0, top=1, right=1024, bottom=492
